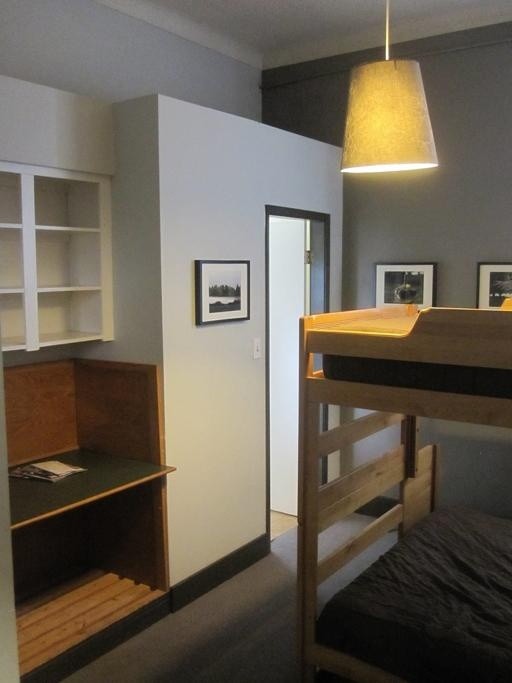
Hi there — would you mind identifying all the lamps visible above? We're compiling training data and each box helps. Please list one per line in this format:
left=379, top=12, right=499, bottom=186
left=341, top=0, right=440, bottom=174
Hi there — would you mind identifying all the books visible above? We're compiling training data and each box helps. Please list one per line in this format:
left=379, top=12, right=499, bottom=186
left=8, top=460, right=86, bottom=483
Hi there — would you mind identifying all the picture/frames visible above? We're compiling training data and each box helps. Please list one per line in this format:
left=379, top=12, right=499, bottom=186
left=194, top=260, right=250, bottom=325
left=375, top=262, right=437, bottom=309
left=476, top=262, right=512, bottom=311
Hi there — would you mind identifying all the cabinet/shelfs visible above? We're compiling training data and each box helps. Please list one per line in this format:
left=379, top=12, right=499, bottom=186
left=1, top=161, right=115, bottom=353
left=3, top=358, right=176, bottom=679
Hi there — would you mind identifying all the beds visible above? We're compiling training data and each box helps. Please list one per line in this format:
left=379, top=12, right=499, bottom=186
left=299, top=304, right=512, bottom=678
